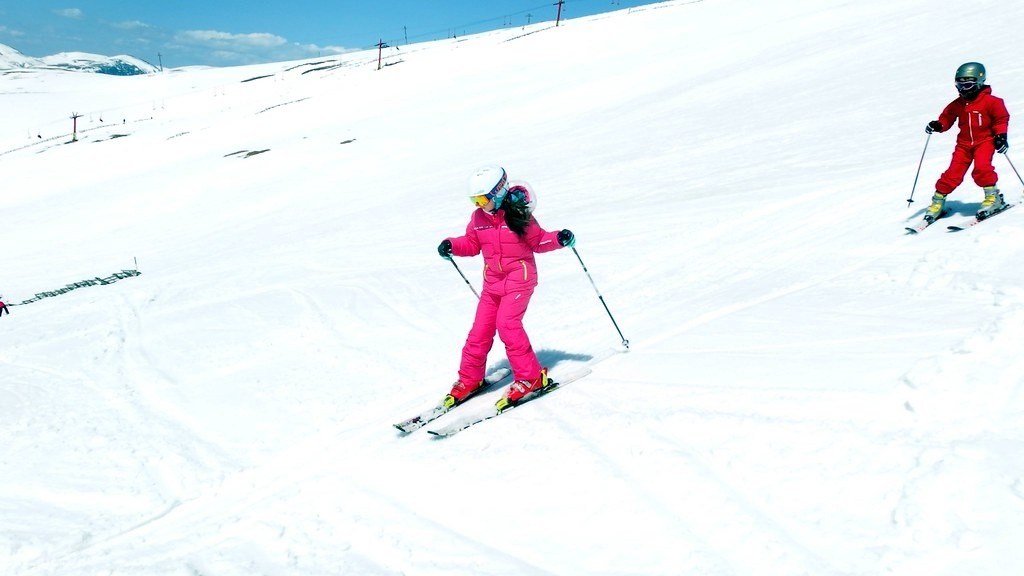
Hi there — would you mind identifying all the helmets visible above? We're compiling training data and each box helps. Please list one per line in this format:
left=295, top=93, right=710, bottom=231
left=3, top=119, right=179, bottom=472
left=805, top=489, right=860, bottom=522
left=468, top=164, right=507, bottom=210
left=955, top=62, right=985, bottom=99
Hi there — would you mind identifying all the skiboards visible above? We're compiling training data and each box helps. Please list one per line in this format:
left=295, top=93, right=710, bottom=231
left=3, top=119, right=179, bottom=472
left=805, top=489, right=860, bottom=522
left=393, top=366, right=559, bottom=436
left=905, top=198, right=1022, bottom=233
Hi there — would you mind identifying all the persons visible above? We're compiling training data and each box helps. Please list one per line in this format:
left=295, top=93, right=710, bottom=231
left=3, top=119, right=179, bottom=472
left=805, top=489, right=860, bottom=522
left=437, top=161, right=576, bottom=413
left=921, top=62, right=1012, bottom=221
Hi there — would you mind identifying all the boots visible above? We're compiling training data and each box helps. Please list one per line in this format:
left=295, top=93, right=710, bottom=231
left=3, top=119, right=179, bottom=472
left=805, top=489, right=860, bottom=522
left=923, top=190, right=946, bottom=224
left=496, top=366, right=548, bottom=409
left=444, top=372, right=490, bottom=406
left=976, top=184, right=1009, bottom=220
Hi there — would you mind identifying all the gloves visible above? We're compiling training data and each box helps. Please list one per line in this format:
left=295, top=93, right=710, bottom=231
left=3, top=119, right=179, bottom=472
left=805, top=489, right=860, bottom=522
left=925, top=120, right=942, bottom=134
left=438, top=240, right=454, bottom=260
left=557, top=229, right=576, bottom=248
left=994, top=133, right=1009, bottom=154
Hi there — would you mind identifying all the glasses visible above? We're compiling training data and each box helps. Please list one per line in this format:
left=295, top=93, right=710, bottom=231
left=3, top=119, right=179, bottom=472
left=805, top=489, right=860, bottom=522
left=467, top=195, right=490, bottom=207
left=955, top=82, right=976, bottom=91
